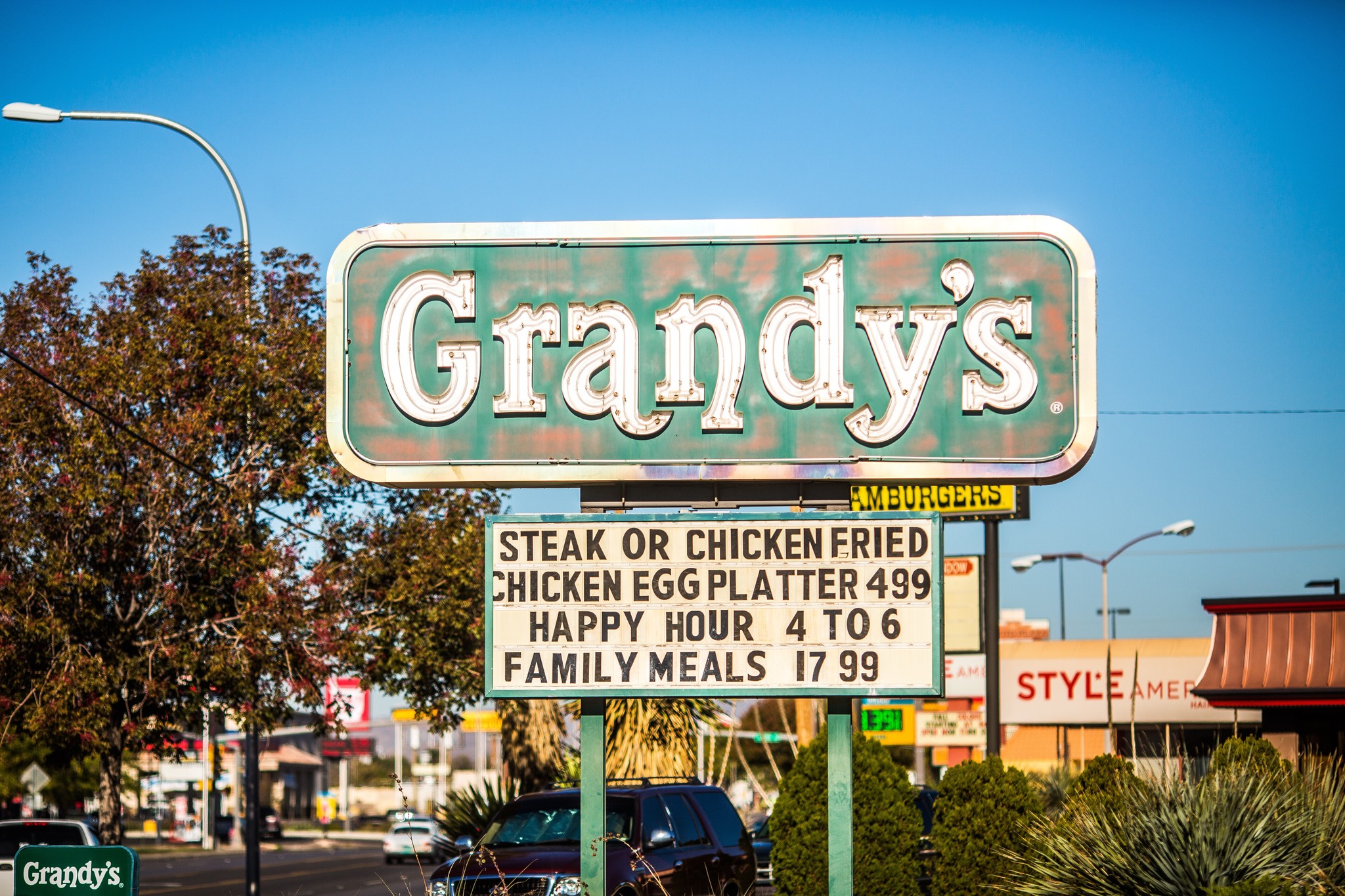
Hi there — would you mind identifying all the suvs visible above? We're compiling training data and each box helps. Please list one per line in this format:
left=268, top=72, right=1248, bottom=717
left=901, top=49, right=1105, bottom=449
left=428, top=774, right=757, bottom=896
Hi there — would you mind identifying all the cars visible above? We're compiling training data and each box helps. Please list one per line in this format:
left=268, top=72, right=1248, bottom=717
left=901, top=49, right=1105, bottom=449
left=0, top=817, right=104, bottom=895
left=382, top=813, right=446, bottom=863
left=751, top=814, right=775, bottom=862
left=217, top=805, right=284, bottom=841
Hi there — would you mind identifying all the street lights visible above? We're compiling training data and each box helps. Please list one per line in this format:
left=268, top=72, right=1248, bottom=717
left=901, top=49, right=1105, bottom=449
left=1096, top=608, right=1131, bottom=641
left=0, top=99, right=267, bottom=896
left=1010, top=520, right=1197, bottom=761
